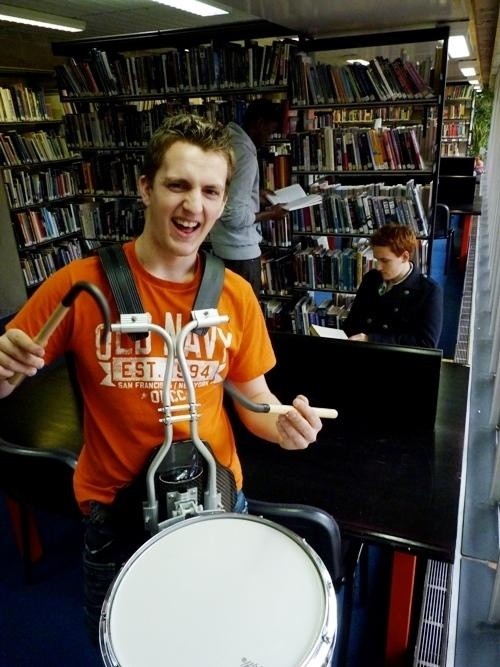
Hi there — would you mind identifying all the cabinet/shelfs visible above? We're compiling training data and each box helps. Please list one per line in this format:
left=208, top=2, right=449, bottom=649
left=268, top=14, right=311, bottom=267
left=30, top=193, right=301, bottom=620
left=51, top=19, right=289, bottom=334
left=289, top=26, right=449, bottom=339
left=0, top=67, right=82, bottom=311
left=440, top=89, right=476, bottom=157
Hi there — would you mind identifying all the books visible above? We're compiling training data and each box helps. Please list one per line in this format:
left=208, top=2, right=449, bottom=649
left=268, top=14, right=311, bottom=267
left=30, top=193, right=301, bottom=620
left=444, top=82, right=476, bottom=158
left=1, top=35, right=475, bottom=118
left=3, top=114, right=167, bottom=301
left=261, top=119, right=440, bottom=337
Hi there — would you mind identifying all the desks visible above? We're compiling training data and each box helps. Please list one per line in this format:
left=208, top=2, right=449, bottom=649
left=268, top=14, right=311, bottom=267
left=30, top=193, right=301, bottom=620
left=436, top=196, right=481, bottom=275
left=0, top=311, right=470, bottom=667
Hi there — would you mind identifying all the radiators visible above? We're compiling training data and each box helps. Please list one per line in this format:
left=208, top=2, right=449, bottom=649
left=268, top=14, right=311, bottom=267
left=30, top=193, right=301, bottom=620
left=453, top=215, right=478, bottom=364
left=413, top=558, right=450, bottom=667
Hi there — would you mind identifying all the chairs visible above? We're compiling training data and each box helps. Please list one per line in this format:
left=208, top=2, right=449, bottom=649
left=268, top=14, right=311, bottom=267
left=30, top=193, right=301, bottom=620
left=437, top=204, right=456, bottom=275
left=0, top=439, right=90, bottom=524
left=245, top=497, right=369, bottom=667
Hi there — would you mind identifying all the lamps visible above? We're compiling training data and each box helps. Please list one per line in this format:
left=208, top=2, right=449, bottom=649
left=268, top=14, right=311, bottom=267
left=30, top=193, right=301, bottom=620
left=434, top=20, right=483, bottom=93
left=343, top=56, right=370, bottom=66
left=0, top=4, right=86, bottom=33
left=150, top=0, right=231, bottom=17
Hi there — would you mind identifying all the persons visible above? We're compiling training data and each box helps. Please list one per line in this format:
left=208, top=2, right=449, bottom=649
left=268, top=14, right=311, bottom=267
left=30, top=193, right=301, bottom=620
left=210, top=101, right=288, bottom=303
left=0, top=114, right=323, bottom=648
left=342, top=224, right=443, bottom=348
